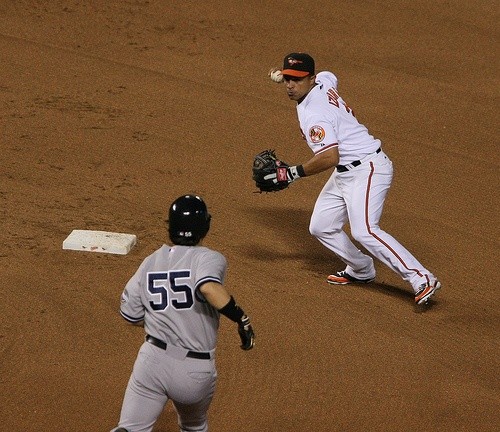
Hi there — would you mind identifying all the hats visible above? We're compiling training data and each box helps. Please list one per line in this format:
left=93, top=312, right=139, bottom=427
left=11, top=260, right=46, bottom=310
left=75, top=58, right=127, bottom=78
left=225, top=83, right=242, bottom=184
left=278, top=52, right=315, bottom=77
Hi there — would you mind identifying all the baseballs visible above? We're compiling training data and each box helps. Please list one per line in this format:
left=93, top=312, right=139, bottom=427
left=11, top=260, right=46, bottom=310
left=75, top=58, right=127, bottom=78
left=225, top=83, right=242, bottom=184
left=271, top=70, right=283, bottom=82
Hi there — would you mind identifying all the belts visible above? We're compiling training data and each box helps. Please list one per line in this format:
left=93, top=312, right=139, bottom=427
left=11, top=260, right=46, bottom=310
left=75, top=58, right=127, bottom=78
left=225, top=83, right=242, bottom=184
left=336, top=147, right=381, bottom=173
left=146, top=334, right=210, bottom=360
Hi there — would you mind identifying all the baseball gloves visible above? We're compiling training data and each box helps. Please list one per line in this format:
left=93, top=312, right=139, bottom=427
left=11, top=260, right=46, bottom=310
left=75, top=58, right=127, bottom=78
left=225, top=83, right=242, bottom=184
left=253, top=150, right=297, bottom=193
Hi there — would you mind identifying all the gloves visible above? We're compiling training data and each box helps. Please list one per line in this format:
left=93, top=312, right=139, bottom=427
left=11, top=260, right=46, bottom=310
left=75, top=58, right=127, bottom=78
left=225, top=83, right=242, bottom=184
left=238, top=316, right=255, bottom=351
left=253, top=166, right=295, bottom=193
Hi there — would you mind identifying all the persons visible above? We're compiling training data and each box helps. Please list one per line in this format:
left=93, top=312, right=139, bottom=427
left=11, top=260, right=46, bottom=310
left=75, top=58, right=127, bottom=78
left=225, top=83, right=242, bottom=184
left=109, top=195, right=255, bottom=432
left=257, top=52, right=441, bottom=306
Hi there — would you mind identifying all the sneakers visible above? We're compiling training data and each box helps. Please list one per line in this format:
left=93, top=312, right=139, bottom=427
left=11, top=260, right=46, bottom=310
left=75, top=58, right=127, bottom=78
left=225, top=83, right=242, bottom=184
left=414, top=278, right=441, bottom=305
left=327, top=270, right=376, bottom=285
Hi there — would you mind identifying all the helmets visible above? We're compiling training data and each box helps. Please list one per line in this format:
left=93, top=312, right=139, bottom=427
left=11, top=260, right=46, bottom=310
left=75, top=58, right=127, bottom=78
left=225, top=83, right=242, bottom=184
left=168, top=194, right=211, bottom=245
